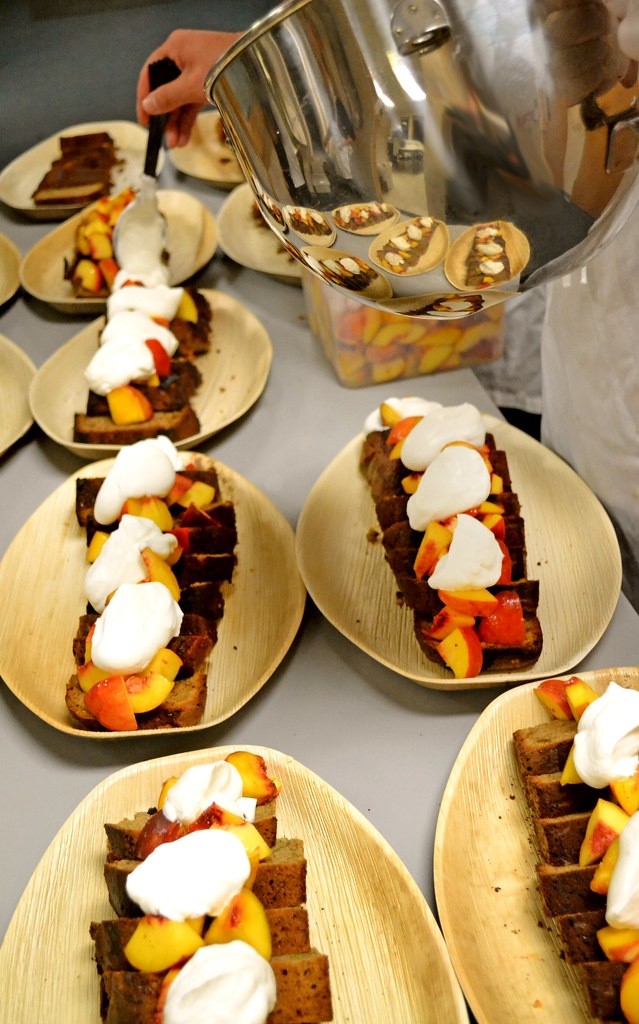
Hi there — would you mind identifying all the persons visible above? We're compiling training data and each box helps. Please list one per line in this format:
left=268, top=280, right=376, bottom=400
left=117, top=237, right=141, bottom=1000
left=139, top=1, right=637, bottom=593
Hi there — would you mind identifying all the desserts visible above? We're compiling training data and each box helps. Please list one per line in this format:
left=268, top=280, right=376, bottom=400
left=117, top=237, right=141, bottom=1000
left=31, top=132, right=333, bottom=1024
left=253, top=191, right=513, bottom=319
left=356, top=398, right=639, bottom=1024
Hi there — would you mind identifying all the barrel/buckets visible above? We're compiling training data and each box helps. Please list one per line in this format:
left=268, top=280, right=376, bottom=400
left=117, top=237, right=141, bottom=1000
left=201, top=0, right=638, bottom=319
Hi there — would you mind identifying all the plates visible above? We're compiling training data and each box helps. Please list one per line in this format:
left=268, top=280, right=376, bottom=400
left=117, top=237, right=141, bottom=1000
left=430, top=666, right=639, bottom=1024
left=2, top=742, right=471, bottom=1023
left=216, top=177, right=305, bottom=280
left=0, top=229, right=25, bottom=309
left=1, top=117, right=165, bottom=220
left=27, top=287, right=269, bottom=458
left=0, top=332, right=41, bottom=463
left=17, top=187, right=220, bottom=316
left=0, top=450, right=307, bottom=737
left=169, top=106, right=249, bottom=183
left=259, top=192, right=533, bottom=318
left=296, top=425, right=623, bottom=689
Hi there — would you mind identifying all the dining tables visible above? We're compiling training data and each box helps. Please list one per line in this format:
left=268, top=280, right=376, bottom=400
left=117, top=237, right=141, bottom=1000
left=0, top=149, right=637, bottom=1023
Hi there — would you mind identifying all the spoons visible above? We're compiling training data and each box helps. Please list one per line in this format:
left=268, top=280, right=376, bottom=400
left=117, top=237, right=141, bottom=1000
left=112, top=55, right=184, bottom=271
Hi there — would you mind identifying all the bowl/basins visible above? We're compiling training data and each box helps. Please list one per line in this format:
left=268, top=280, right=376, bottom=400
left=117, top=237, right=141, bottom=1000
left=300, top=263, right=506, bottom=392
